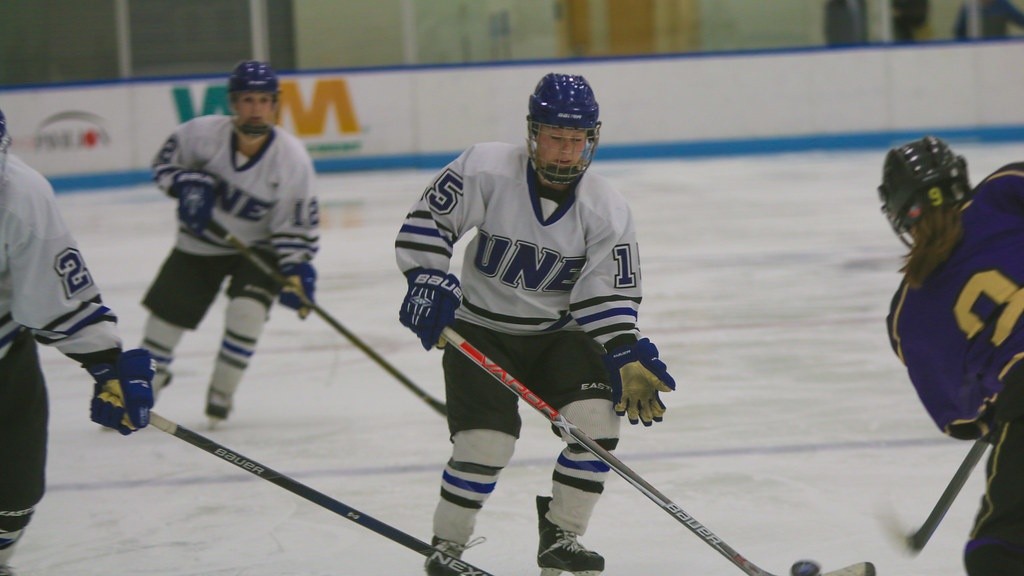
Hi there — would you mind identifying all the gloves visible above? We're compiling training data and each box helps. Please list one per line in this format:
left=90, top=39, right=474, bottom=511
left=611, top=339, right=678, bottom=423
left=396, top=272, right=463, bottom=351
left=85, top=346, right=162, bottom=436
left=170, top=172, right=225, bottom=229
left=280, top=263, right=323, bottom=323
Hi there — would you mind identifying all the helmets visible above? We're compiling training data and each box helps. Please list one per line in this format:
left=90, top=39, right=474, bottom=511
left=880, top=134, right=974, bottom=233
left=525, top=74, right=600, bottom=185
left=227, top=60, right=281, bottom=96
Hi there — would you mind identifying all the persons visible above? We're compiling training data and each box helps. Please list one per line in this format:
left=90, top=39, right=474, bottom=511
left=140, top=61, right=318, bottom=418
left=953, top=0, right=1024, bottom=39
left=878, top=136, right=1024, bottom=576
left=0, top=112, right=157, bottom=576
left=395, top=73, right=675, bottom=576
left=823, top=0, right=927, bottom=44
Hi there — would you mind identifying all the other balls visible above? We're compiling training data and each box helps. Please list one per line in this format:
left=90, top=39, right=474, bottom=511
left=791, top=561, right=820, bottom=576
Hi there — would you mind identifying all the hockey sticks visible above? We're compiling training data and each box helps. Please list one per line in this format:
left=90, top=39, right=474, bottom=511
left=440, top=326, right=877, bottom=576
left=874, top=440, right=990, bottom=558
left=148, top=410, right=494, bottom=576
left=205, top=218, right=448, bottom=418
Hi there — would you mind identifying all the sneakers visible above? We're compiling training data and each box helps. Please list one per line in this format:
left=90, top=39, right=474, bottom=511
left=533, top=494, right=608, bottom=575
left=425, top=537, right=464, bottom=576
left=203, top=385, right=237, bottom=430
